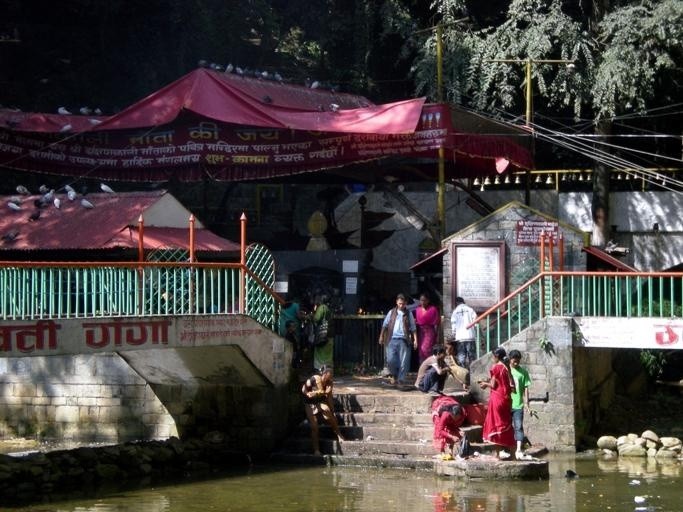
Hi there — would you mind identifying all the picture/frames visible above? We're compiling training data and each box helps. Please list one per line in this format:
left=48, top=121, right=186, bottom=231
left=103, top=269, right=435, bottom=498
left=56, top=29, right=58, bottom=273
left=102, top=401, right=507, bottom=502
left=450, top=239, right=507, bottom=318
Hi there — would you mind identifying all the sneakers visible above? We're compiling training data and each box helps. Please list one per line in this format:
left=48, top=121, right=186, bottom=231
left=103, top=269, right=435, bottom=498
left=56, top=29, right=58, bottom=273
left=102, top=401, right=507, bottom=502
left=498, top=450, right=510, bottom=459
left=428, top=391, right=441, bottom=396
left=514, top=452, right=531, bottom=461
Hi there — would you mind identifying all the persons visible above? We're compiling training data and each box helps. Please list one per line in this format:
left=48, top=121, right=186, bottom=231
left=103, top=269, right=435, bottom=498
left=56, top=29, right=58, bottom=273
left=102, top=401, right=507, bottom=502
left=479, top=346, right=517, bottom=459
left=448, top=297, right=479, bottom=370
left=507, top=350, right=531, bottom=462
left=300, top=368, right=345, bottom=454
left=161, top=288, right=242, bottom=313
left=415, top=293, right=439, bottom=364
left=431, top=396, right=466, bottom=460
left=308, top=293, right=335, bottom=373
left=379, top=292, right=417, bottom=387
left=280, top=291, right=304, bottom=352
left=414, top=345, right=453, bottom=396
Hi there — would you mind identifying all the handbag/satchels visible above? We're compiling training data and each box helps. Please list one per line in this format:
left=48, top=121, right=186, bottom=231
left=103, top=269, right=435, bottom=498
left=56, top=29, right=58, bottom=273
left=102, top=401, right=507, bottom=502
left=314, top=321, right=329, bottom=347
